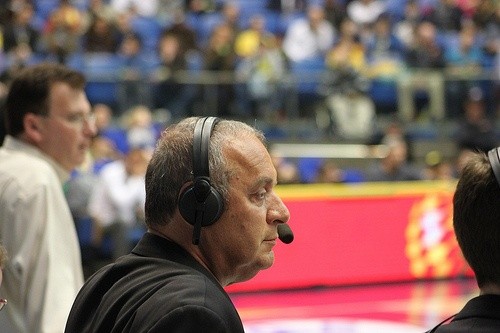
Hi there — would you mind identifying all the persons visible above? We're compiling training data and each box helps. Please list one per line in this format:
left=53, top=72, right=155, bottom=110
left=63, top=116, right=289, bottom=333
left=425, top=147, right=500, bottom=333
left=0, top=64, right=97, bottom=333
left=0, top=0, right=500, bottom=309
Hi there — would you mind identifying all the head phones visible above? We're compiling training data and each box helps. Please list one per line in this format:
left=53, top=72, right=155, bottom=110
left=177, top=116, right=224, bottom=246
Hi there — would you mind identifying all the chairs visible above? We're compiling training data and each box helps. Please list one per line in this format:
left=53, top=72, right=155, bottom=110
left=0, top=0, right=500, bottom=186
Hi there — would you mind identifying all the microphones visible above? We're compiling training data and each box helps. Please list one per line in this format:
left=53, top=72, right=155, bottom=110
left=277, top=223, right=294, bottom=244
